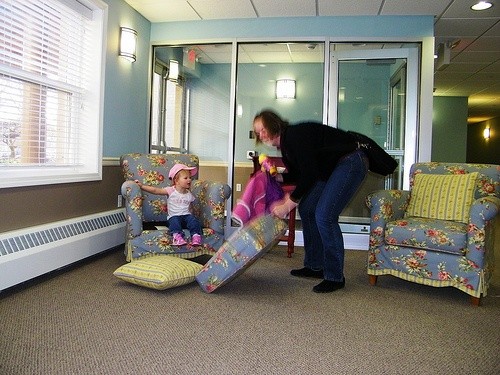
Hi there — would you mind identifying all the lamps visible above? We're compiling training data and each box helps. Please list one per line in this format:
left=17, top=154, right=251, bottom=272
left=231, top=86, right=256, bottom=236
left=483, top=128, right=490, bottom=139
left=274, top=79, right=297, bottom=101
left=168, top=59, right=180, bottom=83
left=118, top=27, right=138, bottom=63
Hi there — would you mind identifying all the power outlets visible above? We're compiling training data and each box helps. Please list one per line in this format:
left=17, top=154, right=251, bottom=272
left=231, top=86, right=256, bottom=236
left=236, top=184, right=242, bottom=192
left=247, top=151, right=256, bottom=159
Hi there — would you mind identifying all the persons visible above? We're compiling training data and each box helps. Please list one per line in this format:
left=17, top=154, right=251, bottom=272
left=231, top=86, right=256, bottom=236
left=135, top=164, right=203, bottom=246
left=253, top=111, right=370, bottom=293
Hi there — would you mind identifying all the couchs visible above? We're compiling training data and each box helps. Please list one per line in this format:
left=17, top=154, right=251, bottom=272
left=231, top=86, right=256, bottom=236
left=121, top=154, right=232, bottom=262
left=366, top=161, right=500, bottom=305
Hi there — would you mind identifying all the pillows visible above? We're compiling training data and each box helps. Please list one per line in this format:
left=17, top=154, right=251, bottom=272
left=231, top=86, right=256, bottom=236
left=404, top=172, right=480, bottom=225
left=112, top=255, right=204, bottom=290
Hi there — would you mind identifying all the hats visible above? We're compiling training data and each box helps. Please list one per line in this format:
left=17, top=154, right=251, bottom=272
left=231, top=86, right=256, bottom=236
left=168, top=163, right=198, bottom=183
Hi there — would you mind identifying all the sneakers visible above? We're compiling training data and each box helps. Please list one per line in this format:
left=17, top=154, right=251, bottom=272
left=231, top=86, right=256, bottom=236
left=191, top=233, right=203, bottom=247
left=172, top=232, right=188, bottom=246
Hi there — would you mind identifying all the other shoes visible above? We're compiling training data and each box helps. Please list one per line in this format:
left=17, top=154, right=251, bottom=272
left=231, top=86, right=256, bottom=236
left=291, top=267, right=324, bottom=279
left=313, top=277, right=345, bottom=293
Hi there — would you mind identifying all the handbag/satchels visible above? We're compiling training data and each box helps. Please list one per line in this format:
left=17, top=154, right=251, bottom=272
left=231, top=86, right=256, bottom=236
left=346, top=130, right=398, bottom=176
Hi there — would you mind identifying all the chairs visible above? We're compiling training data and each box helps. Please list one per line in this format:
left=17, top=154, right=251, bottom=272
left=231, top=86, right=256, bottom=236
left=249, top=156, right=297, bottom=258
